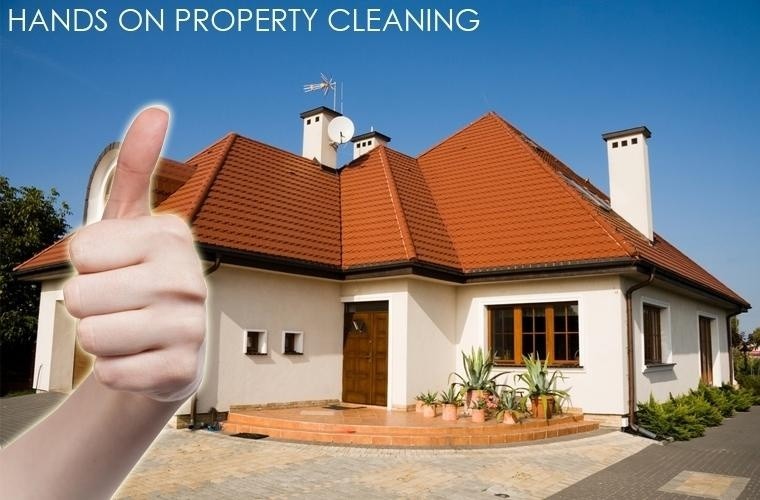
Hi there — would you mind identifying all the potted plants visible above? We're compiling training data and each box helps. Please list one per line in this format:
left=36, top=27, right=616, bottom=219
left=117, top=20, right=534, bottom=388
left=416, top=344, right=575, bottom=426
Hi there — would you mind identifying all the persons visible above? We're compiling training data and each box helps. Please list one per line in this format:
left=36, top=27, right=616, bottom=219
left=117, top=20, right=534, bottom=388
left=0, top=104, right=208, bottom=500
